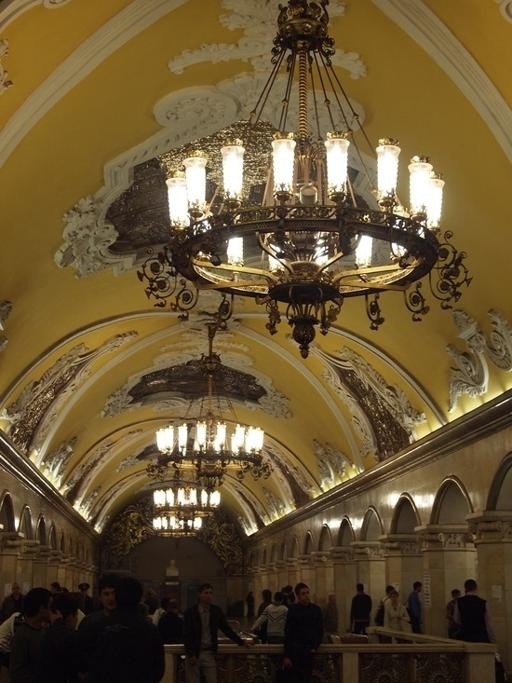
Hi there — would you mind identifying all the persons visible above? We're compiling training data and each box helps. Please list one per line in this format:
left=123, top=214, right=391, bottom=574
left=1, top=577, right=338, bottom=682
left=349, top=579, right=496, bottom=644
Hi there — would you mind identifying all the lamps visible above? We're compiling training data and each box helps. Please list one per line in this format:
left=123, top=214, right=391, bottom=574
left=159, top=0, right=443, bottom=358
left=147, top=459, right=225, bottom=515
left=151, top=504, right=208, bottom=541
left=150, top=320, right=268, bottom=488
